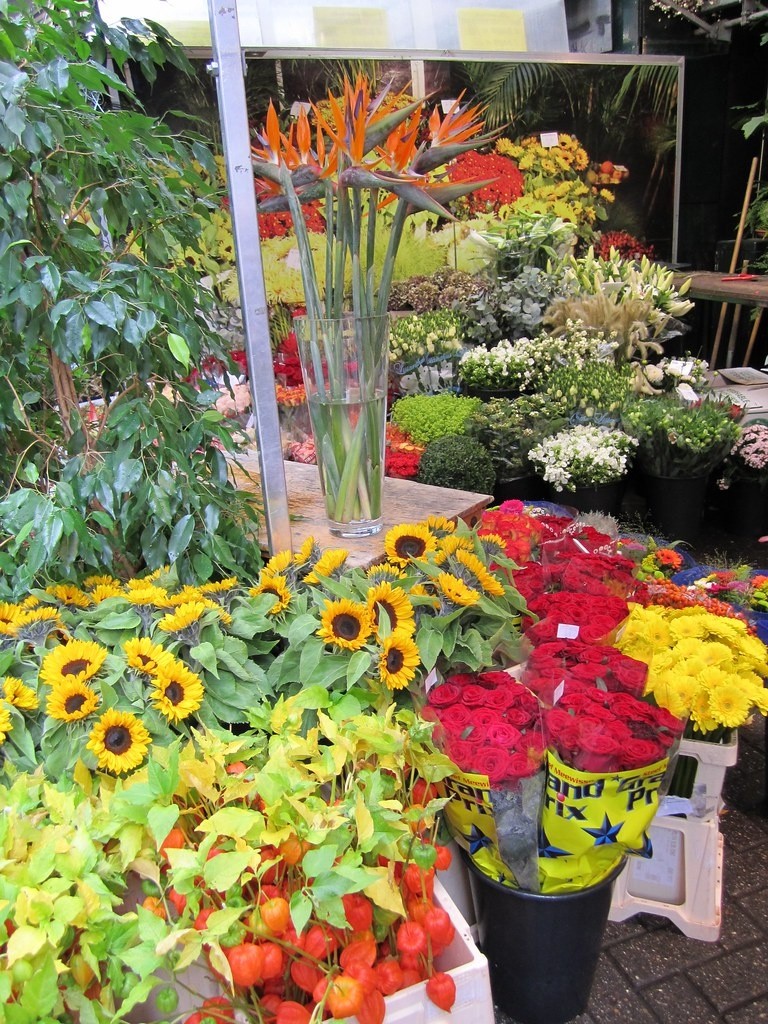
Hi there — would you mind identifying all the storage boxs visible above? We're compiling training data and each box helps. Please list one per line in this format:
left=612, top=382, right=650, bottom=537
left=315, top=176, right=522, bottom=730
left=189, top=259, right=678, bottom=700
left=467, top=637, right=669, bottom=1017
left=608, top=817, right=724, bottom=942
left=677, top=729, right=738, bottom=823
left=219, top=873, right=496, bottom=1024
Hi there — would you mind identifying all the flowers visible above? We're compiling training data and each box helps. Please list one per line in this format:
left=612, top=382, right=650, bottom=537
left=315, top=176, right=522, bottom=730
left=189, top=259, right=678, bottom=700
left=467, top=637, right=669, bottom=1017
left=0, top=72, right=768, bottom=1024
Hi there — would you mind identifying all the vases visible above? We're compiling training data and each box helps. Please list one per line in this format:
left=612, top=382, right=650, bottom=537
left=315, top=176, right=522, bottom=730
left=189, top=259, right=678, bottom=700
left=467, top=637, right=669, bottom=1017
left=723, top=479, right=768, bottom=537
left=490, top=475, right=538, bottom=508
left=456, top=844, right=628, bottom=1024
left=548, top=481, right=620, bottom=518
left=475, top=388, right=530, bottom=401
left=644, top=470, right=712, bottom=533
left=290, top=312, right=389, bottom=538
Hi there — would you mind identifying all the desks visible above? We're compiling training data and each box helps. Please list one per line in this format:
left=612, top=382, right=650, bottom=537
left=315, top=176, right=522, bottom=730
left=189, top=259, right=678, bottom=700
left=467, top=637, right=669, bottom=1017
left=228, top=455, right=495, bottom=573
